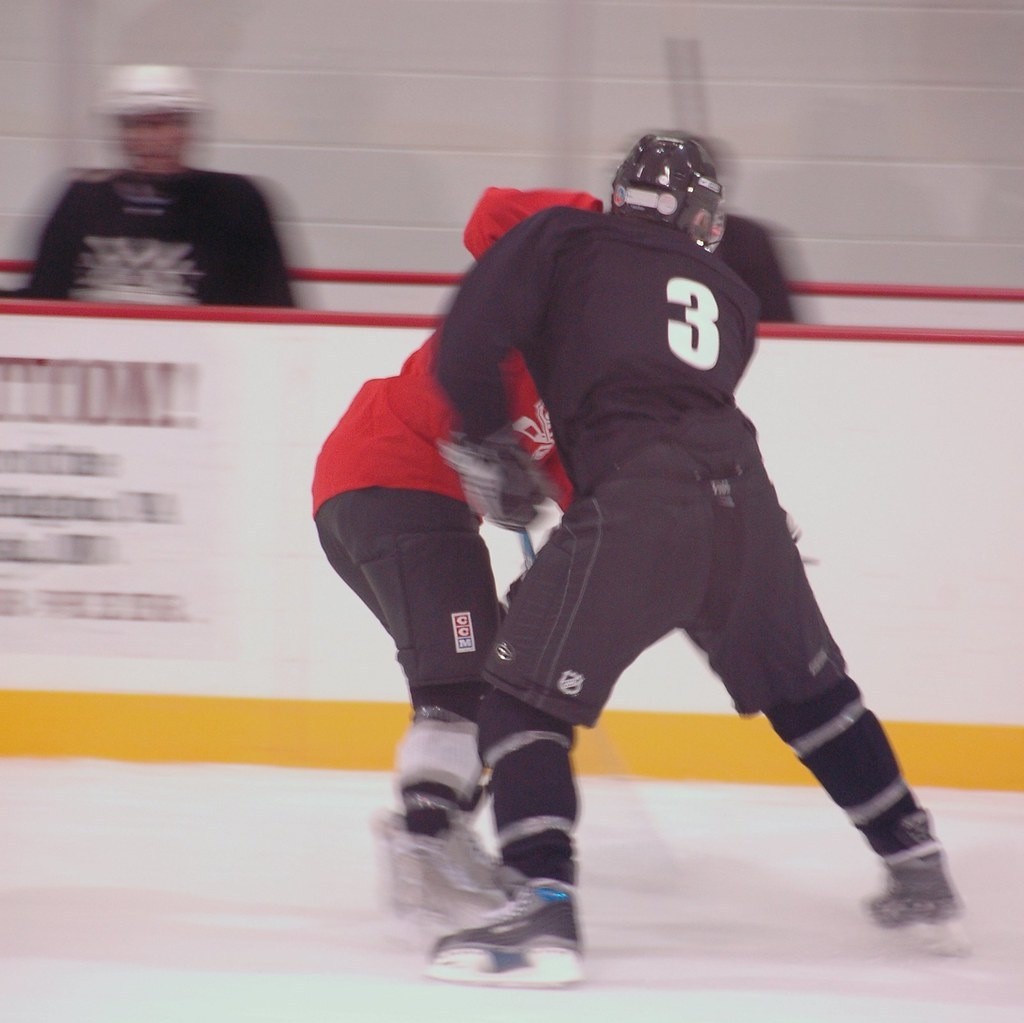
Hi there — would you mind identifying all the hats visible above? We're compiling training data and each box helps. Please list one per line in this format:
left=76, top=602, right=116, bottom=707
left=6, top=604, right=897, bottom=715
left=101, top=64, right=202, bottom=116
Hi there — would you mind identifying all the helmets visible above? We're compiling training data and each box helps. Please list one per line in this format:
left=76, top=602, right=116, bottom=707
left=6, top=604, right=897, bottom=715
left=612, top=133, right=727, bottom=254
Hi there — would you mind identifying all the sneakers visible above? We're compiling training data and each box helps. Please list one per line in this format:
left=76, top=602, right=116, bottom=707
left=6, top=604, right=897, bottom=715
left=864, top=849, right=981, bottom=956
left=377, top=809, right=515, bottom=909
left=417, top=877, right=583, bottom=984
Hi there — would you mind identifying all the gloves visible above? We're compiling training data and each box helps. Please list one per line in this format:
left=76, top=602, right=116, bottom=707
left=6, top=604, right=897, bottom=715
left=460, top=426, right=545, bottom=528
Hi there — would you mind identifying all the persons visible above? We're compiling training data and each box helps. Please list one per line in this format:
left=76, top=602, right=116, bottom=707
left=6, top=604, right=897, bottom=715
left=421, top=125, right=962, bottom=988
left=645, top=127, right=797, bottom=323
left=0, top=62, right=300, bottom=309
left=313, top=187, right=605, bottom=908
